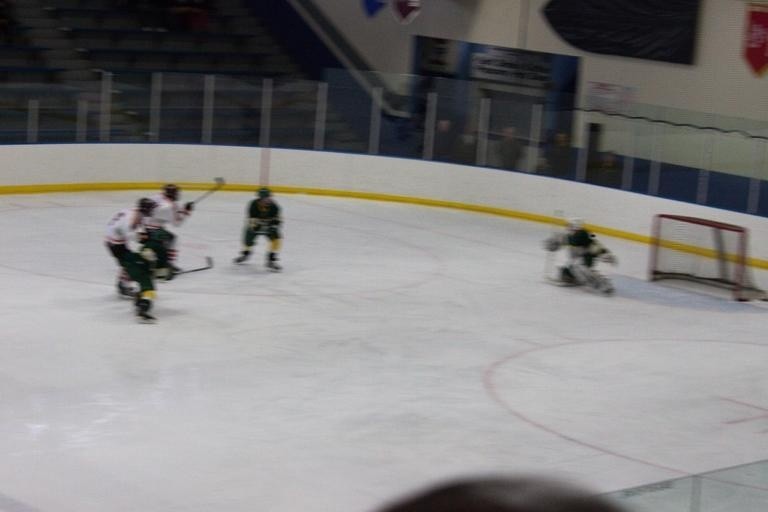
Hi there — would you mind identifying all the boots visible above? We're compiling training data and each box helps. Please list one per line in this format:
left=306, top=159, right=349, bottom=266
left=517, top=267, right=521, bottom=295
left=137, top=299, right=155, bottom=320
left=266, top=253, right=282, bottom=271
left=234, top=249, right=251, bottom=263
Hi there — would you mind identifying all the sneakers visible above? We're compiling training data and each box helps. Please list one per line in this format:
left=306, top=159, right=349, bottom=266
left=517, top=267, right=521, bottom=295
left=121, top=286, right=140, bottom=296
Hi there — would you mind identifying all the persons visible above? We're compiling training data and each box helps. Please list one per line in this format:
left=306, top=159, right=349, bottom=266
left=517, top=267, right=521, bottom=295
left=415, top=116, right=577, bottom=180
left=232, top=186, right=284, bottom=270
left=136, top=183, right=195, bottom=279
left=102, top=198, right=160, bottom=320
left=540, top=226, right=617, bottom=295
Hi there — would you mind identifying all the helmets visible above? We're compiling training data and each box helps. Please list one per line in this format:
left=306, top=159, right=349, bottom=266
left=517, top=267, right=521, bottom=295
left=139, top=199, right=157, bottom=210
left=258, top=189, right=270, bottom=197
left=165, top=184, right=177, bottom=196
left=569, top=217, right=583, bottom=232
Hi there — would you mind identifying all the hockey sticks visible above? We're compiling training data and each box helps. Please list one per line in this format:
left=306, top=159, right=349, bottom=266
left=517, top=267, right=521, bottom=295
left=192, top=177, right=225, bottom=205
left=158, top=256, right=213, bottom=279
